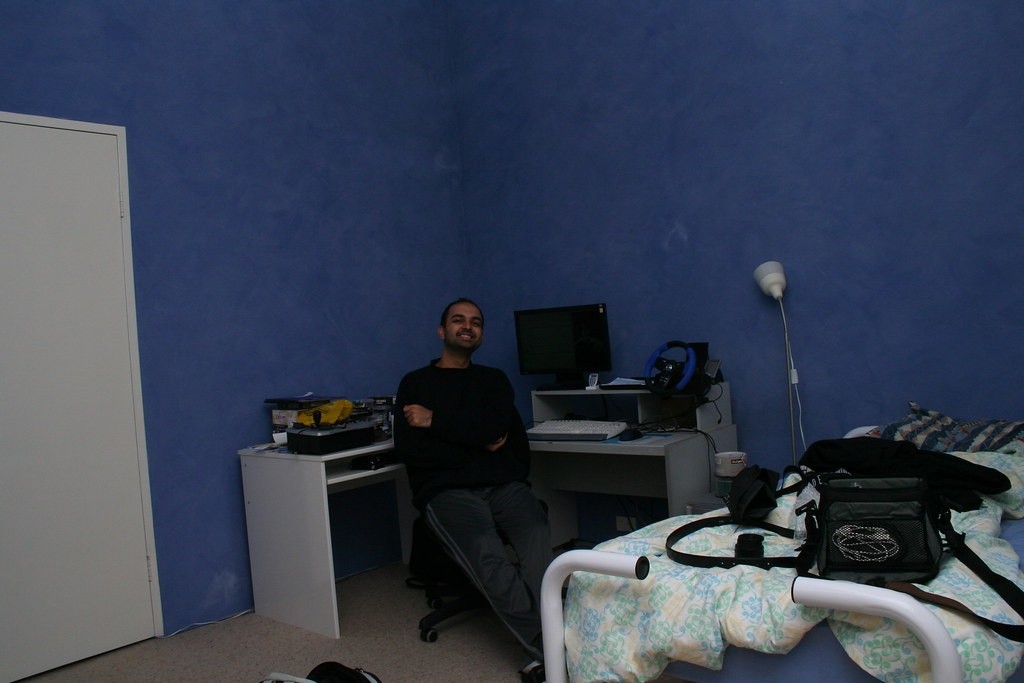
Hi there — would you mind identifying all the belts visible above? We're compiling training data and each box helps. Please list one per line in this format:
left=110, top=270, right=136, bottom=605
left=666, top=517, right=806, bottom=569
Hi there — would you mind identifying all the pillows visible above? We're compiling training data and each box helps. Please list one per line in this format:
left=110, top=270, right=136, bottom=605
left=874, top=399, right=1024, bottom=458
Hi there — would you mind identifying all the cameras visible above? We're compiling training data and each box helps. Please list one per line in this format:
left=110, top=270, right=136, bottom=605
left=733, top=534, right=764, bottom=559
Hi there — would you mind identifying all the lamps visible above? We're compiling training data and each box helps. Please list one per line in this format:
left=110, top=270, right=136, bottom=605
left=751, top=260, right=805, bottom=471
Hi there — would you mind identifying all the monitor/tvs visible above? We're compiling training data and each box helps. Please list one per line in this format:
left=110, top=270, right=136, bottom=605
left=514, top=303, right=612, bottom=391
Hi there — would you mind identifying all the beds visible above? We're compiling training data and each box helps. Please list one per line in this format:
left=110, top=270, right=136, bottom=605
left=538, top=423, right=1024, bottom=683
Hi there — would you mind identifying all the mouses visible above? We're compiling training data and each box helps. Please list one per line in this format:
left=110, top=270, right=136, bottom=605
left=619, top=429, right=643, bottom=441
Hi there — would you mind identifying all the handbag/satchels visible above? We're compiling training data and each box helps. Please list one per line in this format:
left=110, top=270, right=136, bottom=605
left=797, top=496, right=1024, bottom=642
left=729, top=464, right=806, bottom=521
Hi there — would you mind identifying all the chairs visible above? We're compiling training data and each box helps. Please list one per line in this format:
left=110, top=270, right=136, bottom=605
left=418, top=498, right=550, bottom=644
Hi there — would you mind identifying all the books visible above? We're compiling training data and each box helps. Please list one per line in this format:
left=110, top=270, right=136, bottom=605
left=598, top=376, right=648, bottom=390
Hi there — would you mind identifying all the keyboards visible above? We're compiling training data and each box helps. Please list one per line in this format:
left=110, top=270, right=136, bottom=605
left=526, top=419, right=627, bottom=441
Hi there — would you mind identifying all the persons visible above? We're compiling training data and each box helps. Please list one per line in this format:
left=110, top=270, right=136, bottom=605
left=392, top=298, right=553, bottom=683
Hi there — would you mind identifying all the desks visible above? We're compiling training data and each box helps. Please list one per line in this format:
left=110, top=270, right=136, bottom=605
left=235, top=438, right=404, bottom=642
left=529, top=379, right=740, bottom=552
